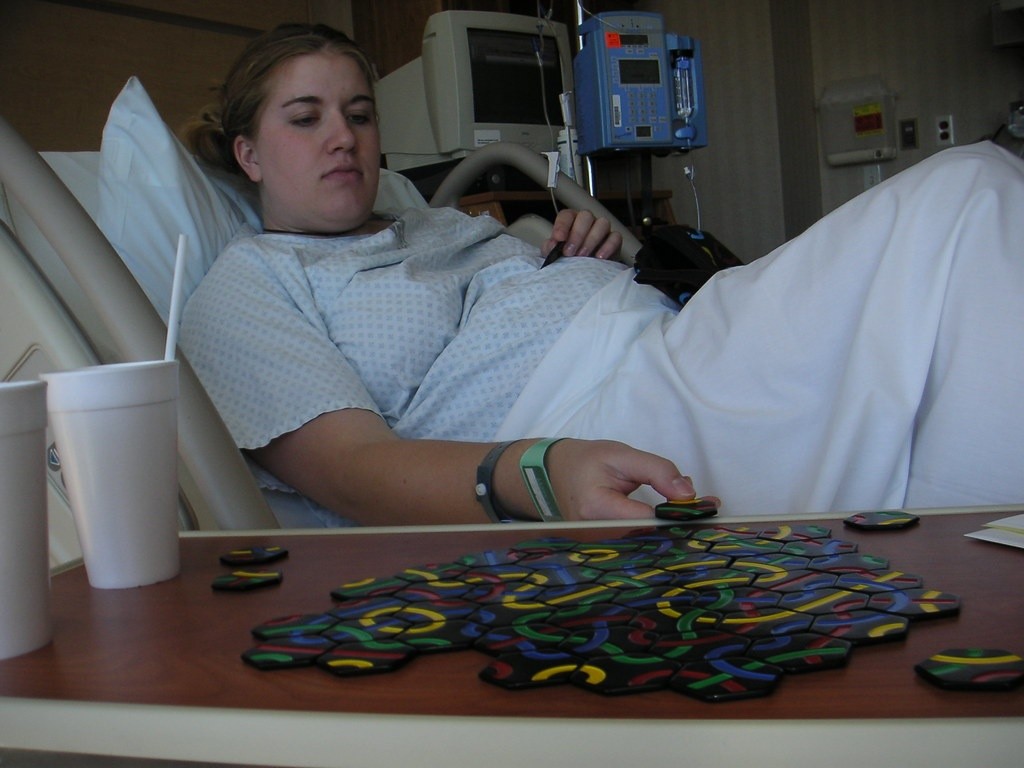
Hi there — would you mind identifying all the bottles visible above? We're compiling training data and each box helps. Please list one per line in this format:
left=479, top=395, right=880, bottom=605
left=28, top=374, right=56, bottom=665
left=557, top=128, right=585, bottom=189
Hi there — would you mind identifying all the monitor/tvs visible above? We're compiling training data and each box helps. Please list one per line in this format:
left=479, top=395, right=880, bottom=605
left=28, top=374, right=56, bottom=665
left=375, top=11, right=575, bottom=172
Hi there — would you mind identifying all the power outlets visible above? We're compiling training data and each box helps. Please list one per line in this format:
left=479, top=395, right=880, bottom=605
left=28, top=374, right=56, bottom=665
left=936, top=115, right=954, bottom=145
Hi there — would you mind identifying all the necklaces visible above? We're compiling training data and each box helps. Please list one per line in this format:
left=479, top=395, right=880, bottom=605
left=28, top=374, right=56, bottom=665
left=263, top=228, right=350, bottom=237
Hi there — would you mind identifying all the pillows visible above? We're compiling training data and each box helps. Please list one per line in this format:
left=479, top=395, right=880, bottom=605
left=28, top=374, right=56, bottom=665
left=88, top=71, right=262, bottom=330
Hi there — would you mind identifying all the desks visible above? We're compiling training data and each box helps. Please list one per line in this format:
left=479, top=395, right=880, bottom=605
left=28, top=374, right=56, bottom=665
left=457, top=189, right=676, bottom=228
left=0, top=512, right=1024, bottom=768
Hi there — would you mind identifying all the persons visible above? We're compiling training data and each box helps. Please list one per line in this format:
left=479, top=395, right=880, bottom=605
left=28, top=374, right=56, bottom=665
left=178, top=22, right=1024, bottom=526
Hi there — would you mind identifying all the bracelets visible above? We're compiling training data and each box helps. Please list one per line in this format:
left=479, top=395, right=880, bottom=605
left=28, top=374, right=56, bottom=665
left=520, top=437, right=573, bottom=523
left=474, top=439, right=521, bottom=522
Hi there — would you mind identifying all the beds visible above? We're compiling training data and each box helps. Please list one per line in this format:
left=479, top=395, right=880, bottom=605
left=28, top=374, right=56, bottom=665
left=0, top=107, right=701, bottom=533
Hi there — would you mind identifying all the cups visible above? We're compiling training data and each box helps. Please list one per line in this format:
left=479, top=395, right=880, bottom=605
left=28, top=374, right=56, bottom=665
left=39, top=359, right=181, bottom=590
left=0, top=379, right=53, bottom=661
